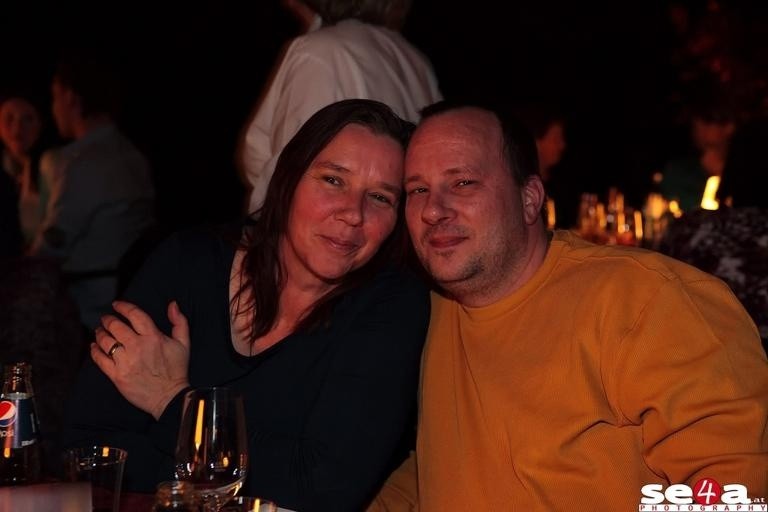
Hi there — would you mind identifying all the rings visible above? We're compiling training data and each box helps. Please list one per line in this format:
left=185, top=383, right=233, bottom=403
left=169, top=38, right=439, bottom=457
left=107, top=343, right=121, bottom=357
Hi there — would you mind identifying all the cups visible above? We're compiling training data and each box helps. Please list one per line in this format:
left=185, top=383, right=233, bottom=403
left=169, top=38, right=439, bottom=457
left=54, top=443, right=129, bottom=511
left=150, top=385, right=277, bottom=511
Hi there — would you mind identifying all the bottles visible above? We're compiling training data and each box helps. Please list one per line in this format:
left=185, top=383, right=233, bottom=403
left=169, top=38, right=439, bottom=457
left=1, top=359, right=47, bottom=486
left=573, top=184, right=682, bottom=243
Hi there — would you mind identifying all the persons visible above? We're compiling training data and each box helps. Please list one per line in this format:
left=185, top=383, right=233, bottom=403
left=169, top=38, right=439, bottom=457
left=657, top=102, right=737, bottom=215
left=0, top=172, right=87, bottom=451
left=37, top=97, right=432, bottom=511
left=518, top=99, right=565, bottom=176
left=367, top=98, right=766, bottom=512
left=1, top=95, right=45, bottom=189
left=28, top=53, right=156, bottom=329
left=662, top=117, right=767, bottom=353
left=242, top=0, right=445, bottom=238
